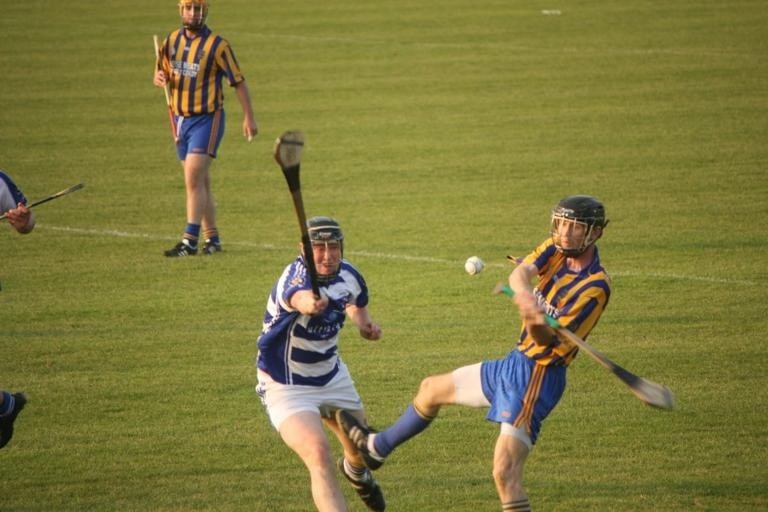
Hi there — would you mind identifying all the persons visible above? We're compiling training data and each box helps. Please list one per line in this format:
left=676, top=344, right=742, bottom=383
left=254, top=217, right=382, bottom=512
left=153, top=0, right=257, bottom=256
left=336, top=195, right=612, bottom=512
left=0, top=171, right=35, bottom=235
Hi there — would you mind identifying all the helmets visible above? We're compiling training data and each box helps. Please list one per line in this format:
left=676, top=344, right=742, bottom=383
left=306, top=217, right=343, bottom=282
left=550, top=195, right=605, bottom=258
left=180, top=0, right=208, bottom=32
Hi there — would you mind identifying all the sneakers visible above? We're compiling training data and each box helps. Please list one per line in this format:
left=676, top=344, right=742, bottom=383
left=165, top=241, right=198, bottom=256
left=203, top=237, right=221, bottom=254
left=335, top=408, right=384, bottom=471
left=338, top=457, right=385, bottom=512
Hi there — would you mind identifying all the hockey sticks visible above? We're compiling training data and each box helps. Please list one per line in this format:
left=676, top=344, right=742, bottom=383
left=273, top=129, right=323, bottom=302
left=493, top=277, right=674, bottom=408
left=0, top=181, right=83, bottom=221
left=152, top=34, right=179, bottom=144
